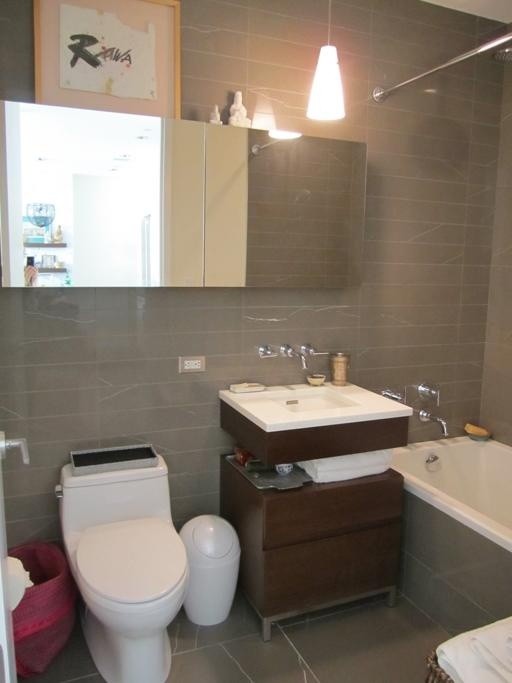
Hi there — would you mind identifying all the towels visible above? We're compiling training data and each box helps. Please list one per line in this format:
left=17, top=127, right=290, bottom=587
left=298, top=449, right=392, bottom=486
left=436, top=617, right=512, bottom=683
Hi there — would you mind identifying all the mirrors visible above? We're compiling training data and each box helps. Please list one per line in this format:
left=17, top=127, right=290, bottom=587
left=2, top=99, right=367, bottom=289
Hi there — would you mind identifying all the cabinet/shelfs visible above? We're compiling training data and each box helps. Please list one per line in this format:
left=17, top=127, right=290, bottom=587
left=23, top=240, right=68, bottom=275
left=219, top=457, right=405, bottom=642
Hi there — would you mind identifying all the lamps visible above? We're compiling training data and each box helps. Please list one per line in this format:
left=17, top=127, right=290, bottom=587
left=304, top=2, right=350, bottom=121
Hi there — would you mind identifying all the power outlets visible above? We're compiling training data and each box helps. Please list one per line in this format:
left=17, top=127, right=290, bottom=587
left=179, top=356, right=205, bottom=373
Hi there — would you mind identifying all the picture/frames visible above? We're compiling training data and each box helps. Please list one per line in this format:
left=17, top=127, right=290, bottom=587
left=34, top=0, right=183, bottom=121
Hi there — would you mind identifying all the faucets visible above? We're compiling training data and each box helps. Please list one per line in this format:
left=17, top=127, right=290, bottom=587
left=418, top=407, right=449, bottom=437
left=299, top=343, right=315, bottom=369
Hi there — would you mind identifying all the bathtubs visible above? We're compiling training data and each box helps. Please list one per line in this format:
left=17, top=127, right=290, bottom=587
left=389, top=436, right=512, bottom=637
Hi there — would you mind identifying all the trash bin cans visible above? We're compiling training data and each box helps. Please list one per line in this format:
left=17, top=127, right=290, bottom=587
left=6, top=540, right=77, bottom=680
left=179, top=514, right=241, bottom=626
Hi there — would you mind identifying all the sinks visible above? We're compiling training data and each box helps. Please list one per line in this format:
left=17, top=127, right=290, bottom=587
left=218, top=382, right=414, bottom=464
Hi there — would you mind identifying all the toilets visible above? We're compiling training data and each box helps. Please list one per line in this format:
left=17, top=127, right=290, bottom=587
left=55, top=444, right=190, bottom=682
